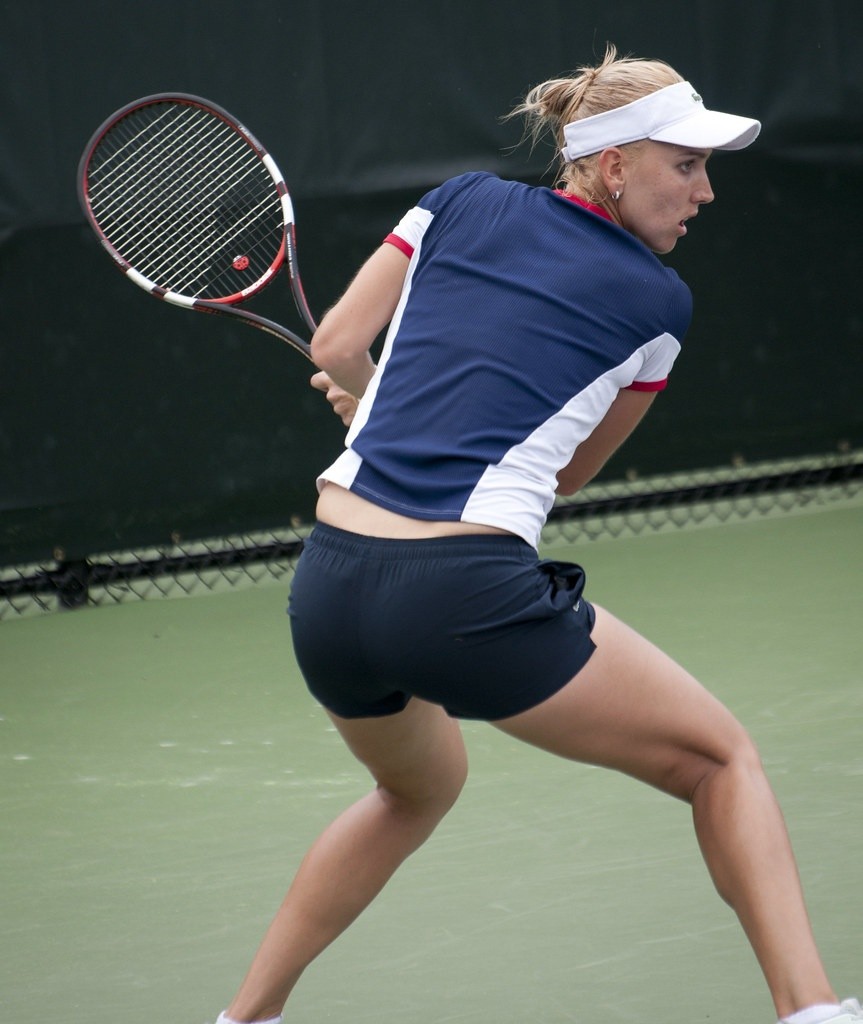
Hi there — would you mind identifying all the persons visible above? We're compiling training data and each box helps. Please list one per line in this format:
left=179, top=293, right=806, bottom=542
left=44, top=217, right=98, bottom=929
left=210, top=46, right=863, bottom=1024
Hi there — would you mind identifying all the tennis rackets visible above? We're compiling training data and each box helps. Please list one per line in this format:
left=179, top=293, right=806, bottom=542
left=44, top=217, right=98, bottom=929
left=72, top=90, right=316, bottom=370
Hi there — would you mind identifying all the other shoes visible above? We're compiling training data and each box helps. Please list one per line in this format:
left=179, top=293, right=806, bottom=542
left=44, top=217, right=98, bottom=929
left=776, top=998, right=863, bottom=1024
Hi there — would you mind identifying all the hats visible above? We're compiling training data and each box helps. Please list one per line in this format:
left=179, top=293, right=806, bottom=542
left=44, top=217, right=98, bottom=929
left=562, top=81, right=761, bottom=163
